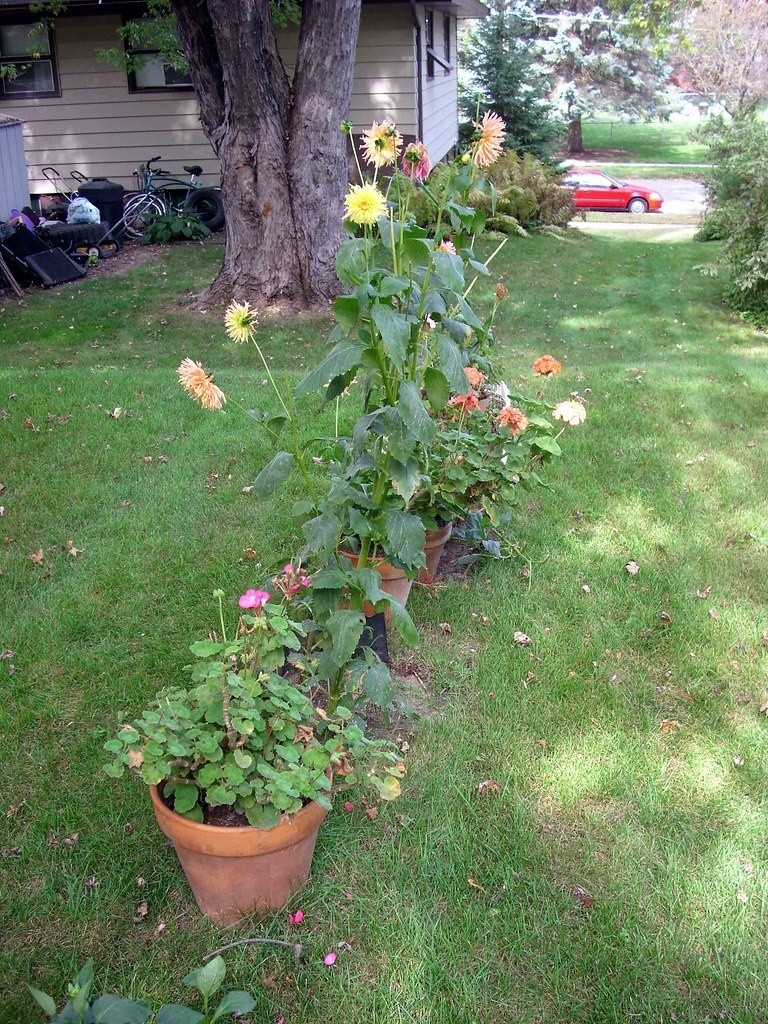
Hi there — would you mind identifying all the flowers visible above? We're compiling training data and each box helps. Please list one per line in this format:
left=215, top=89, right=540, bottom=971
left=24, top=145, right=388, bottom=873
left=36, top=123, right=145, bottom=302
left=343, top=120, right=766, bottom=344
left=103, top=87, right=586, bottom=831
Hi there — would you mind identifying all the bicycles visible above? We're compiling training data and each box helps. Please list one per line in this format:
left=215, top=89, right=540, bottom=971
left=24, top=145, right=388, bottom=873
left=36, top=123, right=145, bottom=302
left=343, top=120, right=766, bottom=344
left=122, top=156, right=224, bottom=237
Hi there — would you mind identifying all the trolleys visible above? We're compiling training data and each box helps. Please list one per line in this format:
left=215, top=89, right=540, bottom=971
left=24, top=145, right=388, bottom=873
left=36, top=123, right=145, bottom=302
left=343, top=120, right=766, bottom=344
left=75, top=188, right=164, bottom=258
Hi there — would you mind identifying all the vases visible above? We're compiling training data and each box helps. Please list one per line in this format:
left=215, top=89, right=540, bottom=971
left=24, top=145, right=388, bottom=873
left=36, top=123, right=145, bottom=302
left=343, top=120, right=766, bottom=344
left=149, top=763, right=331, bottom=929
left=339, top=550, right=414, bottom=620
left=417, top=522, right=452, bottom=584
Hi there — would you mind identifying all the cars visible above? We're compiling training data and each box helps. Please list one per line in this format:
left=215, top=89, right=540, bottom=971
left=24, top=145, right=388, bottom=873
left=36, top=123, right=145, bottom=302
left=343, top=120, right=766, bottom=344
left=558, top=168, right=664, bottom=214
